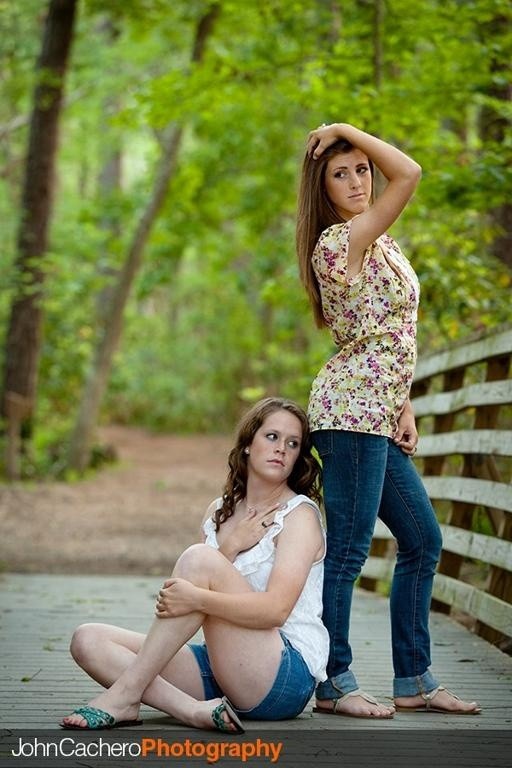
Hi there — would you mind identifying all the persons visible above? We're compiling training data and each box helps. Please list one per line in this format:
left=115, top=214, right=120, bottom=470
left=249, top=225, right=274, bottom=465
left=294, top=122, right=482, bottom=719
left=60, top=398, right=330, bottom=734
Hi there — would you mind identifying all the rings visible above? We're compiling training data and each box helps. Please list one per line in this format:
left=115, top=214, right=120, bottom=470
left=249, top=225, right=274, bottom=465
left=261, top=521, right=268, bottom=528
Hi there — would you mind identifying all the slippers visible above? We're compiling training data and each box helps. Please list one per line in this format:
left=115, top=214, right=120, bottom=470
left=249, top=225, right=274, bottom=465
left=58, top=705, right=144, bottom=731
left=211, top=695, right=245, bottom=735
left=395, top=684, right=482, bottom=714
left=312, top=688, right=394, bottom=719
